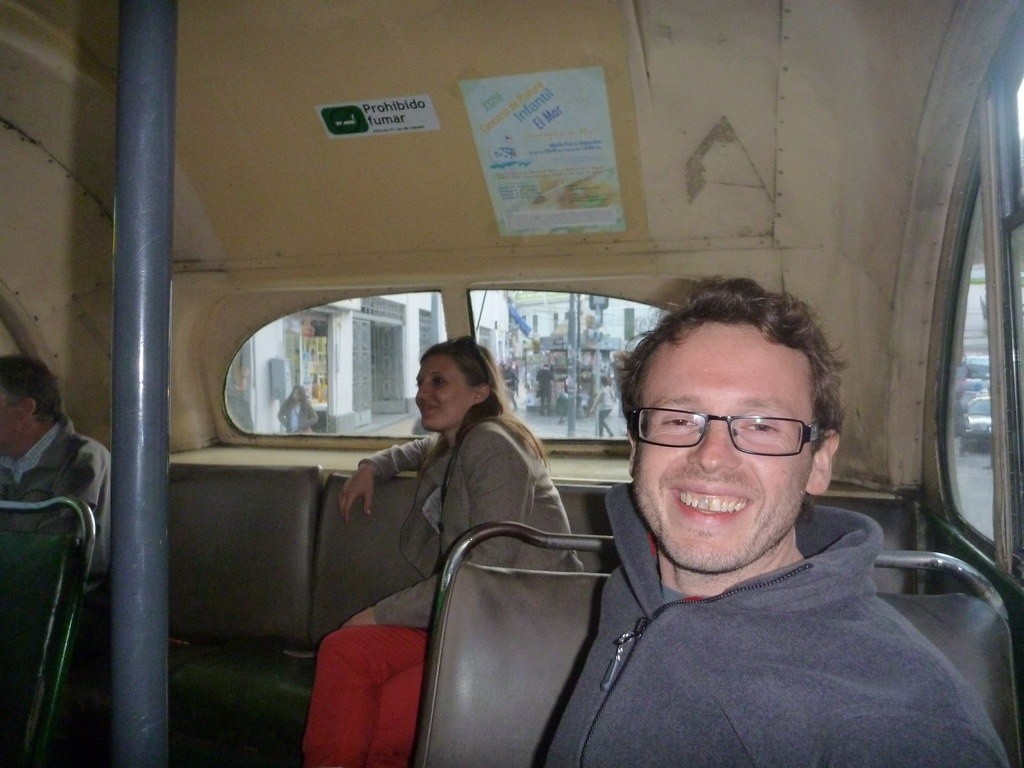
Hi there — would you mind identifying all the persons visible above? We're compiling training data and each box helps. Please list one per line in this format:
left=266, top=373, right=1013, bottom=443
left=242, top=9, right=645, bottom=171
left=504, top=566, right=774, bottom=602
left=0, top=355, right=112, bottom=675
left=546, top=277, right=1013, bottom=768
left=537, top=364, right=553, bottom=416
left=304, top=340, right=582, bottom=768
left=510, top=361, right=519, bottom=396
left=500, top=365, right=518, bottom=411
left=955, top=389, right=971, bottom=457
left=278, top=386, right=320, bottom=433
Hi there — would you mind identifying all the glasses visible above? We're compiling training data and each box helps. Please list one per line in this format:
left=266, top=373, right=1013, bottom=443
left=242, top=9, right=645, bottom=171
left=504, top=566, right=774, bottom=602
left=630, top=407, right=820, bottom=456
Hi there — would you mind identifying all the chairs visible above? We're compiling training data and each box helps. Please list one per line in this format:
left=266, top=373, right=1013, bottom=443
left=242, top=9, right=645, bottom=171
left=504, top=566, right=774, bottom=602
left=165, top=462, right=322, bottom=731
left=409, top=515, right=1024, bottom=766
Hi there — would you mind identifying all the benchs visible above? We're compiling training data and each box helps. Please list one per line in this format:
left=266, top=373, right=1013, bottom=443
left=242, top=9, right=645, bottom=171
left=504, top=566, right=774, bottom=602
left=313, top=470, right=923, bottom=637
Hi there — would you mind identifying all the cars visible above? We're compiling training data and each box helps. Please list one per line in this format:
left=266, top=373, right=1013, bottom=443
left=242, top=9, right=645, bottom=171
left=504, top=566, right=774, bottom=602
left=953, top=354, right=992, bottom=454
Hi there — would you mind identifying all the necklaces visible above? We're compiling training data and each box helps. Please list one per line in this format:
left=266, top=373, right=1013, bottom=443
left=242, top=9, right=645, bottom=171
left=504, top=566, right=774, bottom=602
left=590, top=376, right=618, bottom=437
